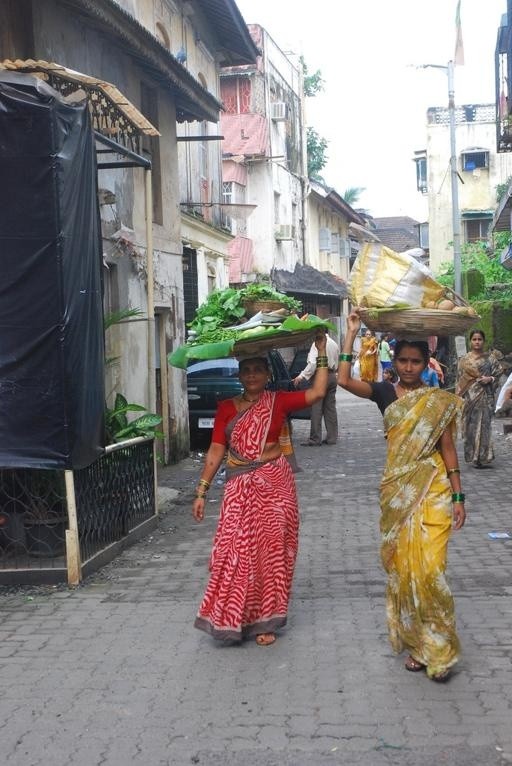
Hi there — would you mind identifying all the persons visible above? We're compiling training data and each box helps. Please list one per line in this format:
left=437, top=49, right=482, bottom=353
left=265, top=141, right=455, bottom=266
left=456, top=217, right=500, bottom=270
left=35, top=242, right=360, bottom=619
left=192, top=331, right=330, bottom=647
left=294, top=328, right=340, bottom=447
left=356, top=329, right=445, bottom=403
left=456, top=329, right=504, bottom=468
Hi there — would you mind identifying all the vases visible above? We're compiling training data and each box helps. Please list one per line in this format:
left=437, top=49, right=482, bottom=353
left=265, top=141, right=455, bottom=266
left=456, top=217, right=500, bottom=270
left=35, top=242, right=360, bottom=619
left=21, top=513, right=69, bottom=560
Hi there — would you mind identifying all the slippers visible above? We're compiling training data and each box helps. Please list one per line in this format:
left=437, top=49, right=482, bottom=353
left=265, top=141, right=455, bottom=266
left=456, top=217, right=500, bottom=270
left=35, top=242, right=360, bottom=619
left=255, top=633, right=276, bottom=646
left=405, top=654, right=423, bottom=672
left=432, top=669, right=452, bottom=682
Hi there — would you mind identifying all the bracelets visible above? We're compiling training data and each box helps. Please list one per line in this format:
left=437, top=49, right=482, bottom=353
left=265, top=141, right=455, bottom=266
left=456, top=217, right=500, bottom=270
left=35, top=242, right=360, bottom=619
left=491, top=377, right=495, bottom=383
left=448, top=468, right=460, bottom=478
left=198, top=479, right=210, bottom=490
left=195, top=493, right=207, bottom=499
left=195, top=487, right=208, bottom=494
left=452, top=492, right=465, bottom=504
left=339, top=353, right=353, bottom=361
left=316, top=356, right=328, bottom=368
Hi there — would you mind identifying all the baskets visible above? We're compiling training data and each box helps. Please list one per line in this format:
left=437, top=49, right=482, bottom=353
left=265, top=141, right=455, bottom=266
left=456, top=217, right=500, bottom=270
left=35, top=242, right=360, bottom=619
left=240, top=297, right=289, bottom=320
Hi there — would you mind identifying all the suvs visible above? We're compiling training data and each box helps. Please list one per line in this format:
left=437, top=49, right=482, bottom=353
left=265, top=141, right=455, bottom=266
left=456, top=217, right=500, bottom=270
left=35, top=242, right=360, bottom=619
left=184, top=345, right=312, bottom=440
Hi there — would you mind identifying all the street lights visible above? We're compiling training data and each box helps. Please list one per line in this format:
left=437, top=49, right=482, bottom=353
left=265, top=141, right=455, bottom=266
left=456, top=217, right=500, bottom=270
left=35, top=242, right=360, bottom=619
left=404, top=58, right=464, bottom=308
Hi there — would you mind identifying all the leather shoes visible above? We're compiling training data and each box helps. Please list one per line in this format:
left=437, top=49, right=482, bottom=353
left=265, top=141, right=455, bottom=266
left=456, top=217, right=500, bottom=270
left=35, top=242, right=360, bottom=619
left=300, top=439, right=336, bottom=446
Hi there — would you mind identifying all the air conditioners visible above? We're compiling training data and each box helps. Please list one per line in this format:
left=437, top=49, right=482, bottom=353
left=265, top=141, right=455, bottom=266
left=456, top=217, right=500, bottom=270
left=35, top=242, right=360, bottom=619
left=276, top=224, right=297, bottom=242
left=271, top=102, right=289, bottom=122
